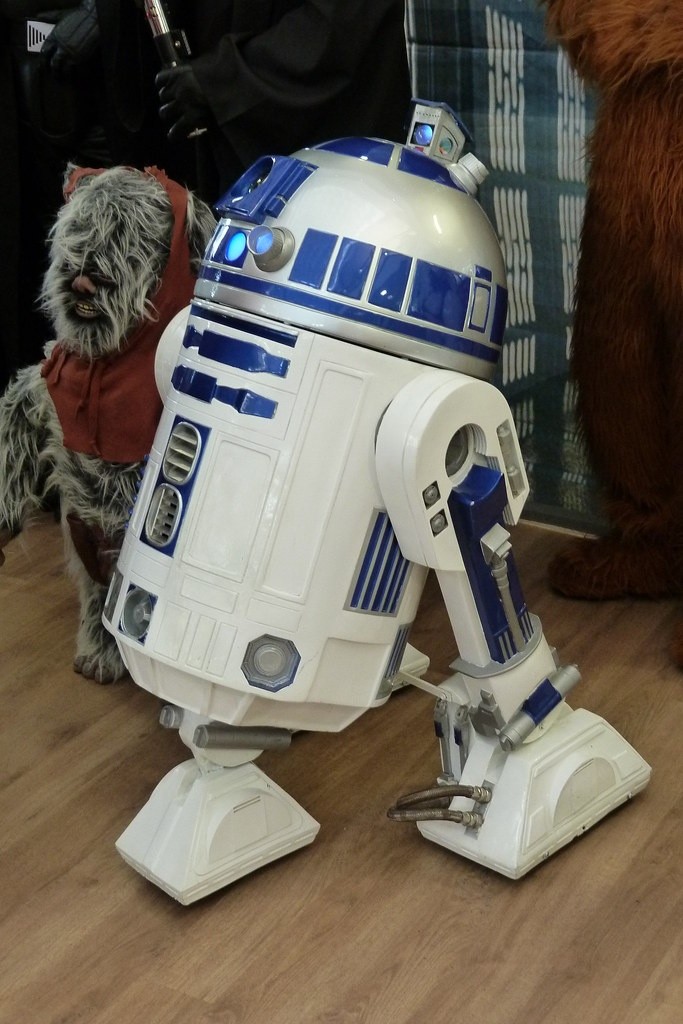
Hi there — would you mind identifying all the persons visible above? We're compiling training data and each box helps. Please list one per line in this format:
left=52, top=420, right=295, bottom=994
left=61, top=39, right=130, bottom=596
left=155, top=0, right=414, bottom=216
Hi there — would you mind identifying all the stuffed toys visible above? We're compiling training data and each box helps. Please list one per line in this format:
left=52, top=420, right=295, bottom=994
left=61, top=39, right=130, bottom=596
left=537, top=0, right=682, bottom=672
left=0, top=159, right=220, bottom=683
